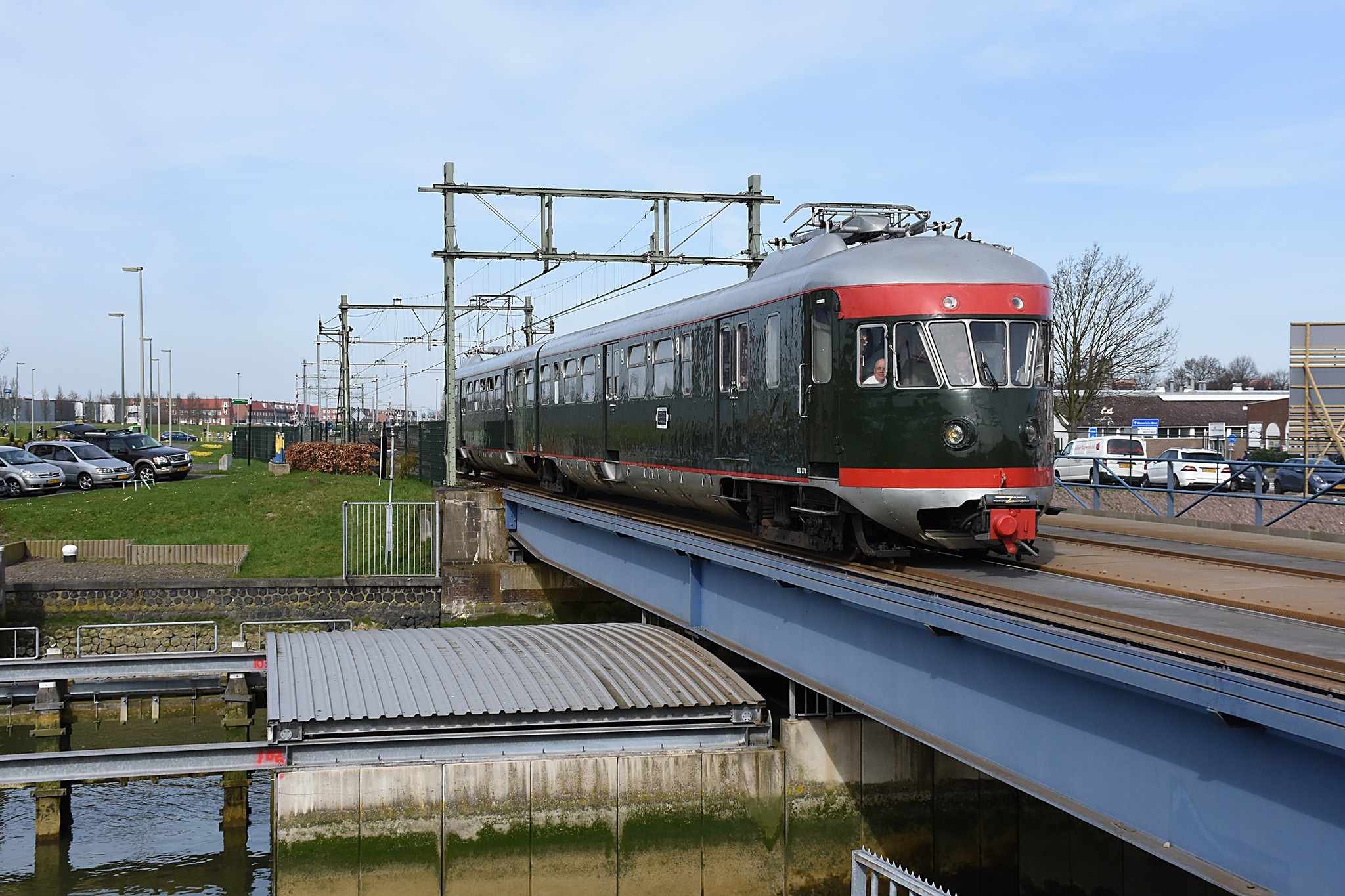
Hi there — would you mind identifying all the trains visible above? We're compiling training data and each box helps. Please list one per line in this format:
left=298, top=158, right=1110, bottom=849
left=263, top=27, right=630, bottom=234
left=442, top=202, right=1066, bottom=570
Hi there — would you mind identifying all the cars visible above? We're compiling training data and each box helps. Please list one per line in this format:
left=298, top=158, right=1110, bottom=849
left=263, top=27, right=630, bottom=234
left=1275, top=458, right=1345, bottom=496
left=15, top=439, right=135, bottom=491
left=127, top=426, right=148, bottom=436
left=0, top=446, right=67, bottom=498
left=160, top=431, right=199, bottom=442
left=1228, top=460, right=1270, bottom=493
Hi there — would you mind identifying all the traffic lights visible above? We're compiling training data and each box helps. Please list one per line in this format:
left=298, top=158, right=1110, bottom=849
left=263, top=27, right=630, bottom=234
left=369, top=421, right=387, bottom=487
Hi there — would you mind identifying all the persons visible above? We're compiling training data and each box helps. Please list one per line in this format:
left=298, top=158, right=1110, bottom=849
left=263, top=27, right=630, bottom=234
left=36, top=425, right=44, bottom=438
left=862, top=358, right=887, bottom=385
left=945, top=346, right=976, bottom=388
left=203, top=426, right=207, bottom=437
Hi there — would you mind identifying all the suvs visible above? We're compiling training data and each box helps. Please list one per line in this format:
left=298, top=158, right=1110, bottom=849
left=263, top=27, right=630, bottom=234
left=51, top=423, right=193, bottom=485
left=1054, top=435, right=1149, bottom=488
left=1141, top=447, right=1232, bottom=493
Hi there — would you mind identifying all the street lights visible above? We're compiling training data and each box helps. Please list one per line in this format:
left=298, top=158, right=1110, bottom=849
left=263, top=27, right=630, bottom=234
left=160, top=349, right=172, bottom=447
left=108, top=312, right=127, bottom=430
left=139, top=337, right=153, bottom=438
left=237, top=371, right=240, bottom=427
left=14, top=362, right=25, bottom=438
left=122, top=265, right=146, bottom=436
left=150, top=358, right=162, bottom=443
left=31, top=367, right=36, bottom=440
